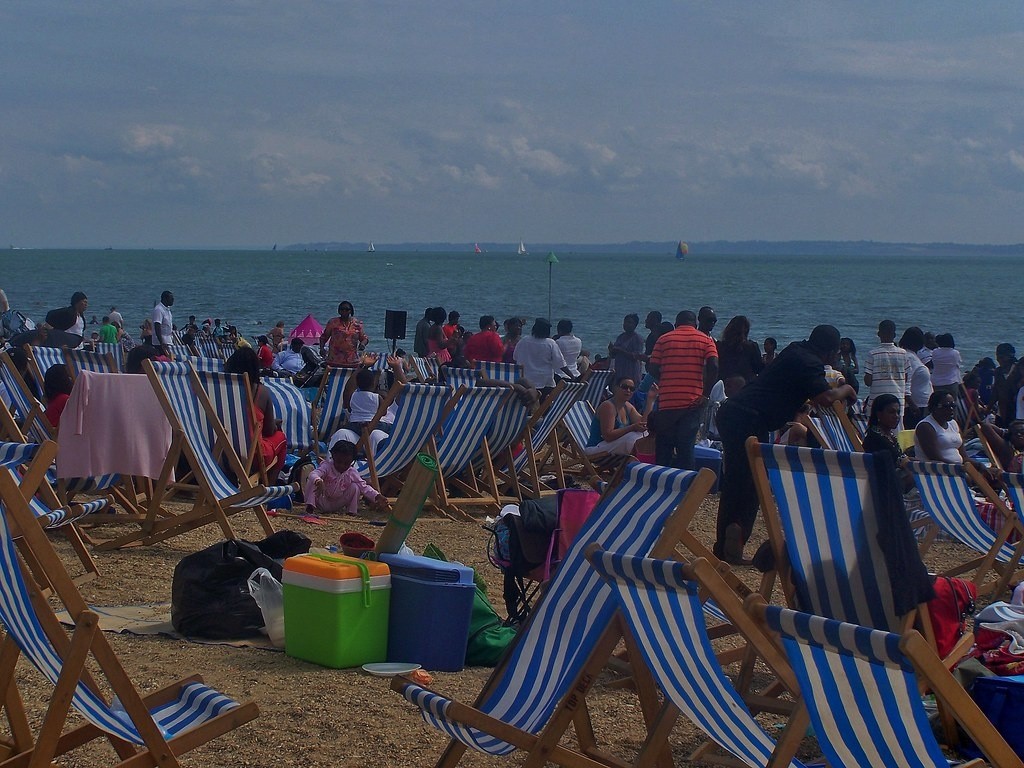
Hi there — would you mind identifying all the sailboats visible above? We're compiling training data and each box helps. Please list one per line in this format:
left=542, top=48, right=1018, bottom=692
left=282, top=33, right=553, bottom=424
left=676, top=240, right=686, bottom=261
left=367, top=240, right=376, bottom=252
left=517, top=237, right=529, bottom=255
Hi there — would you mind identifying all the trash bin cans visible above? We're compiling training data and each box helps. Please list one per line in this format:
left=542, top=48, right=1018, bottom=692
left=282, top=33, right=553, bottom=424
left=266, top=494, right=293, bottom=511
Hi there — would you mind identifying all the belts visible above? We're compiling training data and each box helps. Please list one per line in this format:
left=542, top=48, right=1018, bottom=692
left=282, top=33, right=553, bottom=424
left=723, top=398, right=771, bottom=429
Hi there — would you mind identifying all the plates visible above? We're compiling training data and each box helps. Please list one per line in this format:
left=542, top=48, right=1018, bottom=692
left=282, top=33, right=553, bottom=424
left=362, top=663, right=422, bottom=678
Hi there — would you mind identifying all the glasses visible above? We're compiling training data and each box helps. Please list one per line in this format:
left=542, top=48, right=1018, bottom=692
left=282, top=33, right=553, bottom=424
left=618, top=383, right=636, bottom=392
left=934, top=399, right=955, bottom=410
left=340, top=305, right=352, bottom=311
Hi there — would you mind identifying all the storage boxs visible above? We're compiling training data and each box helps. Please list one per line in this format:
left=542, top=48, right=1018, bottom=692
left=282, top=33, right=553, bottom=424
left=380, top=552, right=475, bottom=675
left=283, top=550, right=393, bottom=670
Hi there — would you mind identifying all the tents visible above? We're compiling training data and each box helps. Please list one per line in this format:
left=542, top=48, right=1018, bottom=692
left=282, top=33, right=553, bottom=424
left=289, top=314, right=325, bottom=348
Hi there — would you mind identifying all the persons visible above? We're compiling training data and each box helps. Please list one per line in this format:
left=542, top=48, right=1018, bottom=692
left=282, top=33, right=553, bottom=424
left=319, top=301, right=369, bottom=366
left=586, top=376, right=659, bottom=457
left=0, top=291, right=410, bottom=518
left=761, top=336, right=779, bottom=364
left=513, top=318, right=576, bottom=403
left=44, top=364, right=75, bottom=425
left=914, top=392, right=1003, bottom=497
left=250, top=335, right=274, bottom=368
left=838, top=338, right=859, bottom=375
left=863, top=393, right=916, bottom=494
left=414, top=306, right=1024, bottom=564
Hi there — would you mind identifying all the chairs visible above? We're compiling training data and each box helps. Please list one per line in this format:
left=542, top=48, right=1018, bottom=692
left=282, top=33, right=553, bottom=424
left=0, top=340, right=1024, bottom=768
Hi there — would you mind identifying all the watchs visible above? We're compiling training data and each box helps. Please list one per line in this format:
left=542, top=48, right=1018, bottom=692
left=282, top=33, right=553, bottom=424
left=537, top=389, right=543, bottom=399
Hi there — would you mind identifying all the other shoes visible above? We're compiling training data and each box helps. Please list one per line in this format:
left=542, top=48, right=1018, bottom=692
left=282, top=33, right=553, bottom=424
left=713, top=555, right=754, bottom=566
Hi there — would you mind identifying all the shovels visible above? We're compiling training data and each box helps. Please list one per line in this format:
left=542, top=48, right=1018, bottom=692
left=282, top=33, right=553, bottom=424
left=266, top=511, right=325, bottom=525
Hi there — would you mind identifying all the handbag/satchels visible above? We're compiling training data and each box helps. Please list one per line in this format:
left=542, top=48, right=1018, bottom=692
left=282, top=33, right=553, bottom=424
left=247, top=568, right=285, bottom=647
left=500, top=512, right=558, bottom=574
left=925, top=572, right=978, bottom=660
left=630, top=429, right=657, bottom=466
